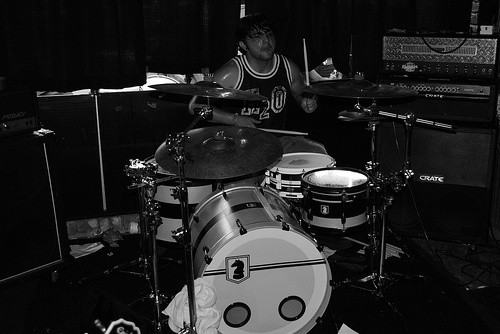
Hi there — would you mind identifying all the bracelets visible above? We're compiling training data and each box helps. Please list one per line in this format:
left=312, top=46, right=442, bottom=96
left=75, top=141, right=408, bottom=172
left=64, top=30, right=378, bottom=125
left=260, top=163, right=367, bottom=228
left=231, top=113, right=241, bottom=127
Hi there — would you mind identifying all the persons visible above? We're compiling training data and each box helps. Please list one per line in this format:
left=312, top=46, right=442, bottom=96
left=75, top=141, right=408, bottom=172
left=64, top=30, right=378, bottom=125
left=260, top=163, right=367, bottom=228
left=189, top=16, right=327, bottom=155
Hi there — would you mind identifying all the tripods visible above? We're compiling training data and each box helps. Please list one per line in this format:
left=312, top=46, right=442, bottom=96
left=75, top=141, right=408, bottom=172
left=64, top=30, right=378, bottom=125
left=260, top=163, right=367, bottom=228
left=76, top=175, right=159, bottom=304
left=331, top=198, right=427, bottom=324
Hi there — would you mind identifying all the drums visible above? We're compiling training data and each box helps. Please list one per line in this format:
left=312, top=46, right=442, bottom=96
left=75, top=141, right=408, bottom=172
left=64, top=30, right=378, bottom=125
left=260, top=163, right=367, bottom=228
left=265, top=151, right=336, bottom=204
left=187, top=183, right=333, bottom=334
left=141, top=174, right=217, bottom=250
left=300, top=166, right=371, bottom=236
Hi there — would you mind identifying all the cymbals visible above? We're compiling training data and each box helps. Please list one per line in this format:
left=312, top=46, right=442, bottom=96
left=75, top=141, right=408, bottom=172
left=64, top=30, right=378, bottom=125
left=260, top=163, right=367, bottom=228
left=148, top=80, right=267, bottom=100
left=155, top=125, right=283, bottom=180
left=301, top=77, right=415, bottom=98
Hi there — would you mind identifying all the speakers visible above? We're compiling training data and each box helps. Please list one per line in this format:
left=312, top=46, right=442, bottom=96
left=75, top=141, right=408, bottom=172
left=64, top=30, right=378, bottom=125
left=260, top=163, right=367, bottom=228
left=0, top=126, right=66, bottom=286
left=374, top=120, right=496, bottom=189
